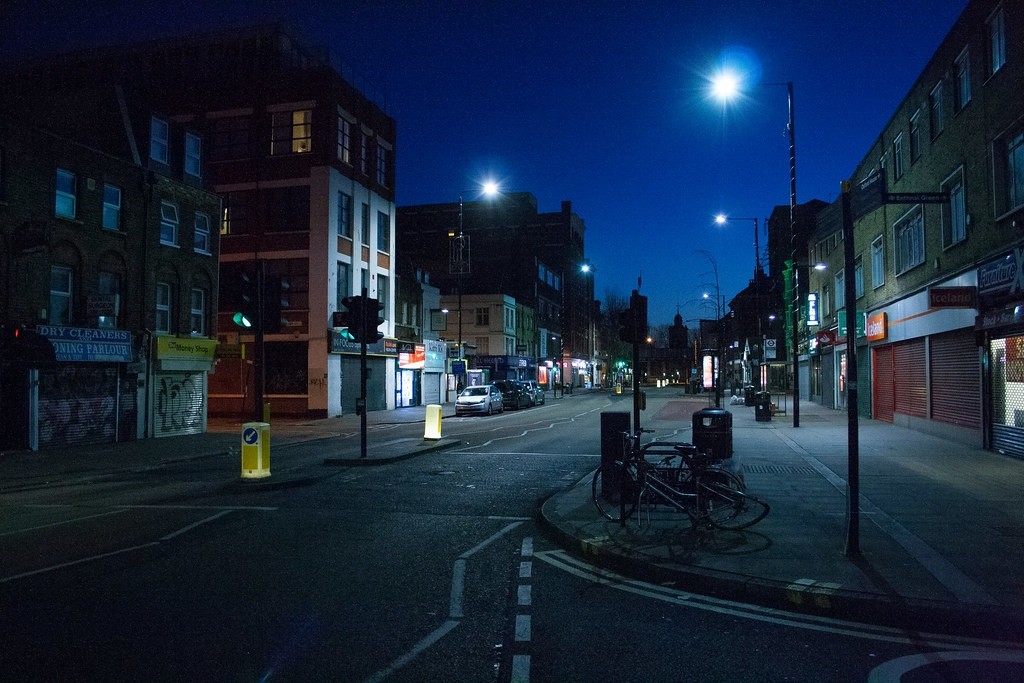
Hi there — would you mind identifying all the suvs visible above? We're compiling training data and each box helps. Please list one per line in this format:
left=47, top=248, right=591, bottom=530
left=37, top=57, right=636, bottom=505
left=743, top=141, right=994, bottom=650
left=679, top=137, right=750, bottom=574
left=519, top=380, right=546, bottom=406
left=489, top=379, right=532, bottom=410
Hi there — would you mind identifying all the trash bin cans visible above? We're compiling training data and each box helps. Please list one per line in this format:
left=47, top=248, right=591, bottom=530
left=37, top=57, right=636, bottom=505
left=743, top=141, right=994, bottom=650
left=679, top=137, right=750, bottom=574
left=691, top=379, right=701, bottom=394
left=744, top=385, right=755, bottom=407
left=755, top=391, right=770, bottom=421
left=692, top=407, right=732, bottom=501
left=616, top=383, right=622, bottom=393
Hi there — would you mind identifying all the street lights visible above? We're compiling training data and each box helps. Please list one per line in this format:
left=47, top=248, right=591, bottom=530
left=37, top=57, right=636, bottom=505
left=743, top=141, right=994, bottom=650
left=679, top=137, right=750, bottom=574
left=716, top=214, right=765, bottom=391
left=458, top=180, right=498, bottom=396
left=709, top=292, right=727, bottom=396
left=709, top=71, right=800, bottom=428
left=572, top=263, right=590, bottom=360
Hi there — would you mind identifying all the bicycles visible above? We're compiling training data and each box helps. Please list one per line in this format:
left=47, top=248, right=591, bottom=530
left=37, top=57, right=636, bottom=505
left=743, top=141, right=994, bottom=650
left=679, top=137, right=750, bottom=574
left=592, top=428, right=772, bottom=537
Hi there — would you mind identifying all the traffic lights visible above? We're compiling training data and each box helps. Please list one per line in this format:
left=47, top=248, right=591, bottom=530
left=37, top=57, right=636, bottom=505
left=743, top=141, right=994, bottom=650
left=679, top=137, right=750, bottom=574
left=377, top=301, right=385, bottom=340
left=278, top=281, right=291, bottom=327
left=240, top=266, right=257, bottom=330
left=618, top=310, right=630, bottom=343
left=13, top=327, right=20, bottom=338
left=618, top=361, right=626, bottom=368
left=342, top=295, right=362, bottom=342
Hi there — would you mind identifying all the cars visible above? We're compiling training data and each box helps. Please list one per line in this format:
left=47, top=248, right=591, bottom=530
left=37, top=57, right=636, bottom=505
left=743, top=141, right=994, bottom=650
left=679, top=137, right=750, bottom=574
left=455, top=385, right=504, bottom=417
